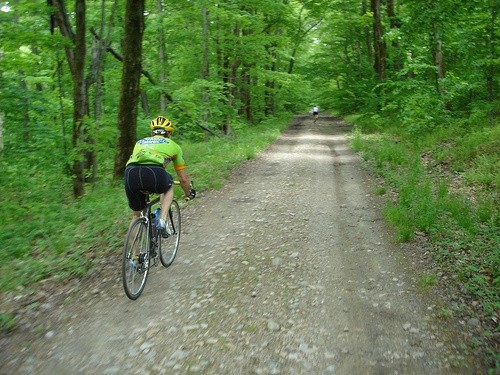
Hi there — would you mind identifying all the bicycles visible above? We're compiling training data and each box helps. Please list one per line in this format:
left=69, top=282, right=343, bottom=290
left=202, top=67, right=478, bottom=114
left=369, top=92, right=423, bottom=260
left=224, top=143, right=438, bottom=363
left=121, top=181, right=196, bottom=301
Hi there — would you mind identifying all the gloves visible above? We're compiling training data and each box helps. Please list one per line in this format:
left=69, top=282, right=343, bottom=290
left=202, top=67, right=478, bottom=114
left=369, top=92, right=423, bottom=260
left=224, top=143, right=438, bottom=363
left=187, top=189, right=196, bottom=200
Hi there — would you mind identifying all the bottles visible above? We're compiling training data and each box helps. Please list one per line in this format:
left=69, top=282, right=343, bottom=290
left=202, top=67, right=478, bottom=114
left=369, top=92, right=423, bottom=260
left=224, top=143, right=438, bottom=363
left=155, top=208, right=161, bottom=228
left=151, top=214, right=157, bottom=237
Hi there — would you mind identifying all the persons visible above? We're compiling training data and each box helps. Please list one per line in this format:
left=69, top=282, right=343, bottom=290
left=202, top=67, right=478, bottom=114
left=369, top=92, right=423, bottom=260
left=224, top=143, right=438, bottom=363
left=124, top=116, right=196, bottom=265
left=312, top=105, right=319, bottom=123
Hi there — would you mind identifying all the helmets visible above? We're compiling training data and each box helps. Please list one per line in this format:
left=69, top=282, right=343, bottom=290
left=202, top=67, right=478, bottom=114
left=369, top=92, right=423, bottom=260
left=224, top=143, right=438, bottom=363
left=150, top=116, right=173, bottom=134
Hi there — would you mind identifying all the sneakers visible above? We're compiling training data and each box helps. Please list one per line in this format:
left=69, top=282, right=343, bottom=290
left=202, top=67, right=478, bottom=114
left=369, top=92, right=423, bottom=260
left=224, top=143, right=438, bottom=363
left=130, top=257, right=142, bottom=268
left=158, top=219, right=171, bottom=236
